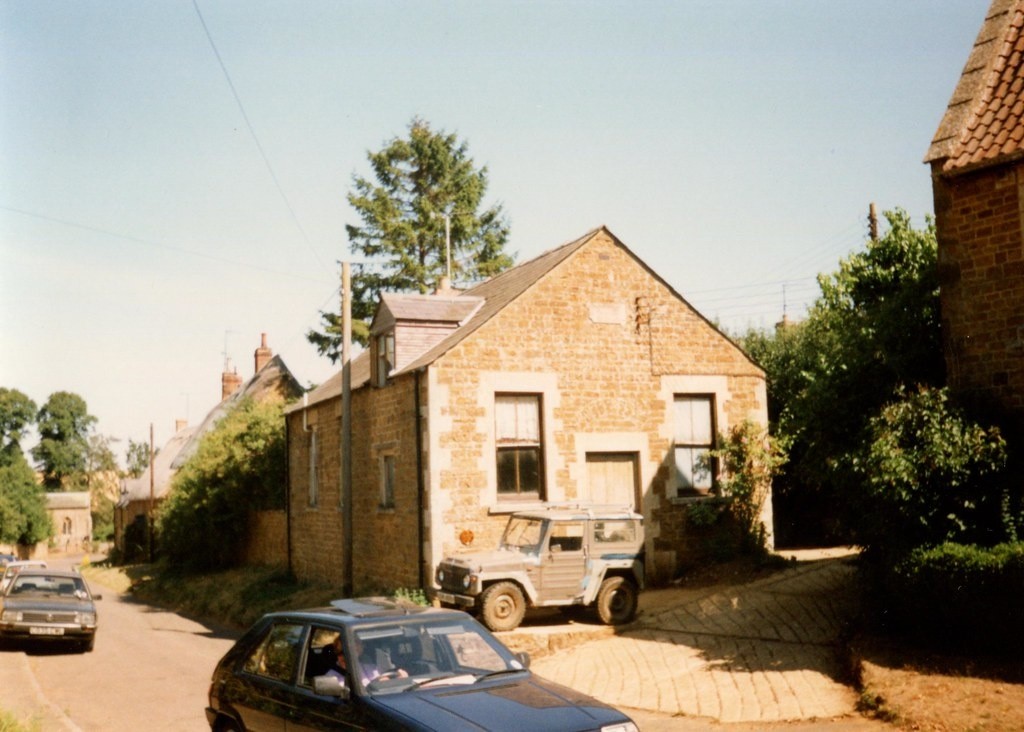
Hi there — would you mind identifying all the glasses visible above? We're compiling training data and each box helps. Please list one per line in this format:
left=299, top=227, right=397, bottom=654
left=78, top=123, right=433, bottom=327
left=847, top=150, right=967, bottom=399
left=337, top=651, right=344, bottom=657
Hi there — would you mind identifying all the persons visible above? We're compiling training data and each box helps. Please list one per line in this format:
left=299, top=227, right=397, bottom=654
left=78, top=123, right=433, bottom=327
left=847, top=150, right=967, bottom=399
left=327, top=631, right=410, bottom=690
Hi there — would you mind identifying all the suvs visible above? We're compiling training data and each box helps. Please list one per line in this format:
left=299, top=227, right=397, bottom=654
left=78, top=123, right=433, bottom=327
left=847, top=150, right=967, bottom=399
left=437, top=508, right=648, bottom=634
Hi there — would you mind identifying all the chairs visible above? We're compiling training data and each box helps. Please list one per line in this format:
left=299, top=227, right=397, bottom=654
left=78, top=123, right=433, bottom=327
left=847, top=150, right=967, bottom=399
left=58, top=584, right=73, bottom=595
left=22, top=584, right=36, bottom=591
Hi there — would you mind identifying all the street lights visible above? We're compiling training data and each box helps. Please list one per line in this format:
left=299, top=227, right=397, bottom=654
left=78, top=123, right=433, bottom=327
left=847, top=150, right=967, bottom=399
left=338, top=255, right=374, bottom=598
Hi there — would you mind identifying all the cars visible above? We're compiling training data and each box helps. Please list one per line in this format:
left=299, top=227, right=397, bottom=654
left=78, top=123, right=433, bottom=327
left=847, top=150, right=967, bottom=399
left=205, top=595, right=640, bottom=732
left=0, top=569, right=102, bottom=653
left=0, top=562, right=54, bottom=593
